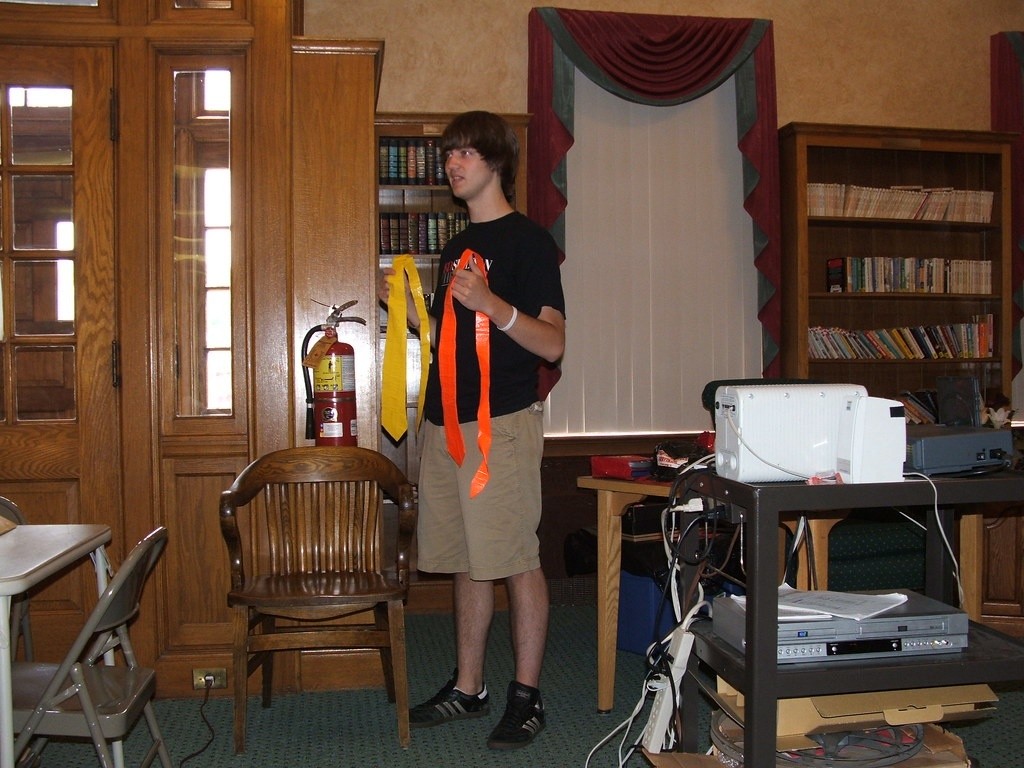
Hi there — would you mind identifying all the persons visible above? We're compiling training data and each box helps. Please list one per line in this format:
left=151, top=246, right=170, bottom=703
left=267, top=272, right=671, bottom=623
left=377, top=109, right=566, bottom=748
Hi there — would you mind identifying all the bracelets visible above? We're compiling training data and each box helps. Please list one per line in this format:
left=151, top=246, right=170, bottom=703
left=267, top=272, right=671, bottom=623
left=497, top=306, right=517, bottom=333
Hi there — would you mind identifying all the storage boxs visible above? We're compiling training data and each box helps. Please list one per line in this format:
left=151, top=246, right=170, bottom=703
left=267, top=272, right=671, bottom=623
left=642, top=672, right=998, bottom=768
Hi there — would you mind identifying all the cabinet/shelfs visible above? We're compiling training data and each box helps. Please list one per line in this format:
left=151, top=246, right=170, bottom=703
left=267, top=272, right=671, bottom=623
left=0, top=0, right=384, bottom=696
left=374, top=111, right=526, bottom=338
left=774, top=121, right=1012, bottom=404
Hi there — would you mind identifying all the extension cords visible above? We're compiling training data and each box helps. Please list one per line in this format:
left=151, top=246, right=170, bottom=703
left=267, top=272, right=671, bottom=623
left=642, top=628, right=695, bottom=753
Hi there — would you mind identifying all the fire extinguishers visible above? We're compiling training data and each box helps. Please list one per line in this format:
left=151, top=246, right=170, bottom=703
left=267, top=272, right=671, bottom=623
left=301, top=298, right=366, bottom=448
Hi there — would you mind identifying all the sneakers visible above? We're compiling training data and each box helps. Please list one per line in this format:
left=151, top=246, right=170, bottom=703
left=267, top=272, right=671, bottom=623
left=409, top=678, right=489, bottom=728
left=487, top=680, right=546, bottom=749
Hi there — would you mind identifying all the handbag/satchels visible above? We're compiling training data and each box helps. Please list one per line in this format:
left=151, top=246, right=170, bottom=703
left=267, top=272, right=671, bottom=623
left=617, top=562, right=671, bottom=656
left=591, top=455, right=653, bottom=480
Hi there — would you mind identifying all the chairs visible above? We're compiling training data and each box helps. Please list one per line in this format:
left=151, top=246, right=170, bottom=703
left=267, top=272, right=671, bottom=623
left=218, top=447, right=415, bottom=753
left=11, top=527, right=174, bottom=768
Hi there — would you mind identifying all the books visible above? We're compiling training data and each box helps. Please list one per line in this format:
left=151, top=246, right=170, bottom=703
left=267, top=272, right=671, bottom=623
left=806, top=181, right=993, bottom=426
left=377, top=138, right=470, bottom=314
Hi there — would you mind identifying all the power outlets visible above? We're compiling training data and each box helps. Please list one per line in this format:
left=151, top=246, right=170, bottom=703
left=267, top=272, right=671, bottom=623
left=191, top=668, right=227, bottom=690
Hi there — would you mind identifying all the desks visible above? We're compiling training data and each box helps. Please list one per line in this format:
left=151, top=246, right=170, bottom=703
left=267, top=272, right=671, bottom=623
left=577, top=465, right=1024, bottom=768
left=0, top=525, right=127, bottom=768
